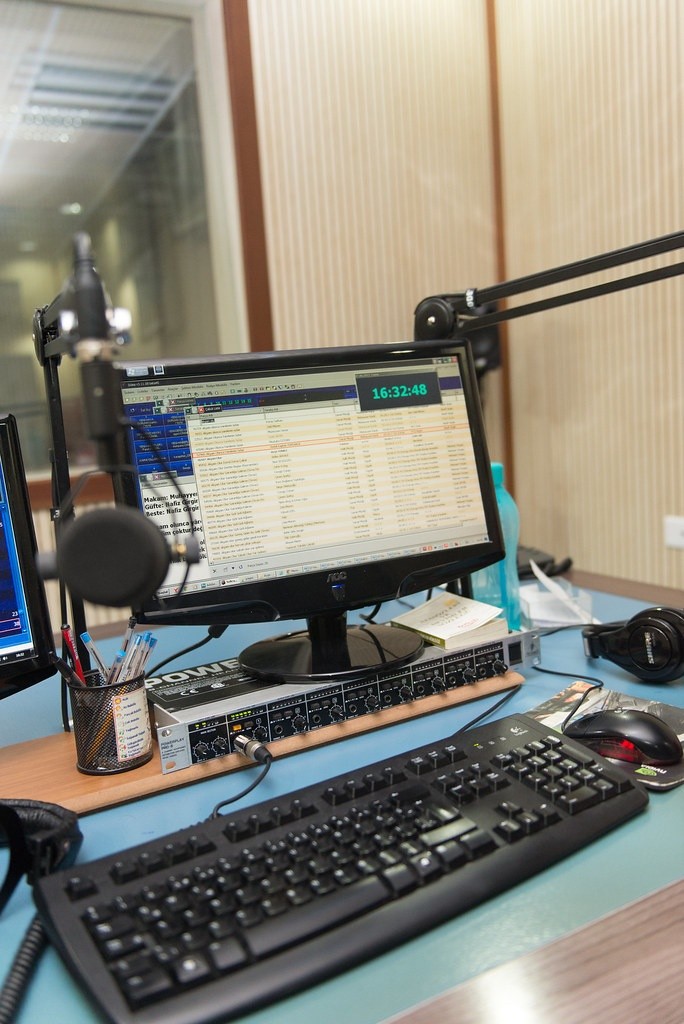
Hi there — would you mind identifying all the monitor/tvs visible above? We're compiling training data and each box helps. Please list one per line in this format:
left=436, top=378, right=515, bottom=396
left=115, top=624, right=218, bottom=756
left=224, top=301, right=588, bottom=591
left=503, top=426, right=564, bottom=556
left=108, top=337, right=506, bottom=683
left=0, top=413, right=57, bottom=698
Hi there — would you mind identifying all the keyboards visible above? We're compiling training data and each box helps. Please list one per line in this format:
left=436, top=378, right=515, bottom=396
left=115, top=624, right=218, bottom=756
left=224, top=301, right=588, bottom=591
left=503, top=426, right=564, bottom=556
left=30, top=712, right=650, bottom=1024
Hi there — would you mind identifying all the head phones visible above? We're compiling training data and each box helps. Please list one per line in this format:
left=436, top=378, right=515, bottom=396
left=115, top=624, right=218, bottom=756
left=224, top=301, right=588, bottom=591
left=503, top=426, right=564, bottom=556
left=582, top=606, right=684, bottom=684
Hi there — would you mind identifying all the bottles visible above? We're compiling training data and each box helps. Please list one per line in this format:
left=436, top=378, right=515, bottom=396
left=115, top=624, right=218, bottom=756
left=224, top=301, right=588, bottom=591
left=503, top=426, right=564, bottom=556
left=470, top=462, right=522, bottom=630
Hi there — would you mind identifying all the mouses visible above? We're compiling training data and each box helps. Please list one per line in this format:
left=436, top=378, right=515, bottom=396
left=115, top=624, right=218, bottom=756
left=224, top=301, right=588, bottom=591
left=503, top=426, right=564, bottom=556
left=564, top=710, right=683, bottom=766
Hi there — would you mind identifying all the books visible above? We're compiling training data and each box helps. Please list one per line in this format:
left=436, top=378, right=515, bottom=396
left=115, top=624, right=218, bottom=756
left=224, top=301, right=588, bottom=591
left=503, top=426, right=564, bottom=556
left=390, top=591, right=508, bottom=651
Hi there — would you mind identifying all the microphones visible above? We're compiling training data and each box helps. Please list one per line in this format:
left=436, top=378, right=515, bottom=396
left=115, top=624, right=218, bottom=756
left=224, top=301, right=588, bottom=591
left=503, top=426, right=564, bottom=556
left=38, top=233, right=200, bottom=614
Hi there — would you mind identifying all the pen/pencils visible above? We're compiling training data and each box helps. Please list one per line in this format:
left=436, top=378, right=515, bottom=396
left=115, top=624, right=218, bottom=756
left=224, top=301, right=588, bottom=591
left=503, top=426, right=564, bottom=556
left=46, top=615, right=157, bottom=769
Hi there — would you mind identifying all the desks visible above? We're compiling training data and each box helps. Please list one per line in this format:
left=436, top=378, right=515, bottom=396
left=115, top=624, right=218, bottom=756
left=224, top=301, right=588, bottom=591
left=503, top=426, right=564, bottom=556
left=0, top=569, right=684, bottom=1024
